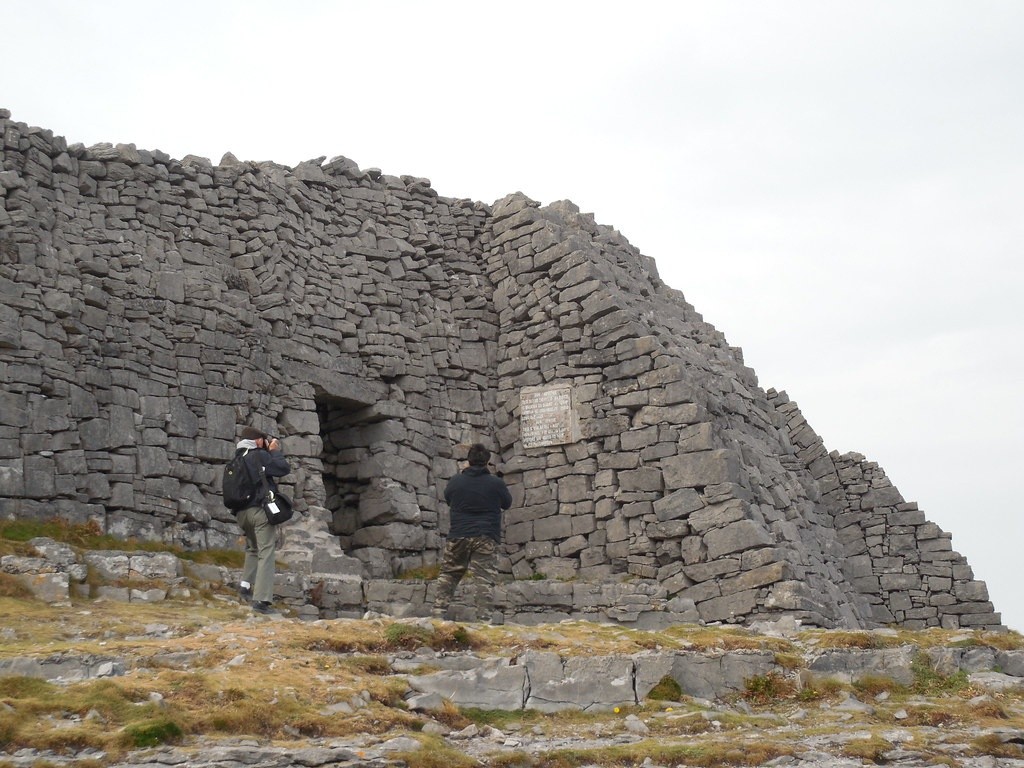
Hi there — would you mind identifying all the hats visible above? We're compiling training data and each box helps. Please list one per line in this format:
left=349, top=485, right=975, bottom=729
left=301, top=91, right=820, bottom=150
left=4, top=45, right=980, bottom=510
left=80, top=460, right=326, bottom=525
left=243, top=427, right=268, bottom=440
left=467, top=443, right=491, bottom=463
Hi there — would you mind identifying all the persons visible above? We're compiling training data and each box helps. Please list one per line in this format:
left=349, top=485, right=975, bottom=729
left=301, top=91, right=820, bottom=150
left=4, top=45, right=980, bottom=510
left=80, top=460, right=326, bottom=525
left=233, top=426, right=290, bottom=614
left=433, top=444, right=512, bottom=623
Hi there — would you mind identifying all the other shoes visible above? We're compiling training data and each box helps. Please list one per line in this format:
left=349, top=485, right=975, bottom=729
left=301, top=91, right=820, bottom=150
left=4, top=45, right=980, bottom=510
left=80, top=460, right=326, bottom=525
left=238, top=586, right=253, bottom=598
left=253, top=601, right=274, bottom=614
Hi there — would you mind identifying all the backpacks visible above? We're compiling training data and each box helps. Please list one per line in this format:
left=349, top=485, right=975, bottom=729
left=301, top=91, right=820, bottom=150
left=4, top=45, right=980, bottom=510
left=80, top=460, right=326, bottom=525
left=222, top=449, right=265, bottom=508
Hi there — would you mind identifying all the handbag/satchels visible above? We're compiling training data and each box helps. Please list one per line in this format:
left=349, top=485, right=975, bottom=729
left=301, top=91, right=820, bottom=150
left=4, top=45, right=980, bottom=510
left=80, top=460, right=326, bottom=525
left=264, top=491, right=293, bottom=525
